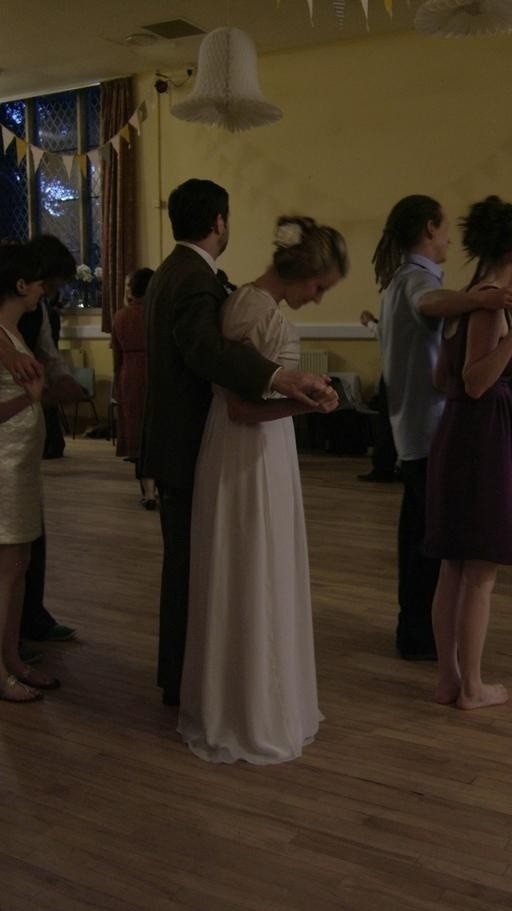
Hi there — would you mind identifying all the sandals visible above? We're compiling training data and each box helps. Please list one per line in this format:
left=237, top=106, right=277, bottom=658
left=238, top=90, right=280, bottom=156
left=0, top=665, right=61, bottom=702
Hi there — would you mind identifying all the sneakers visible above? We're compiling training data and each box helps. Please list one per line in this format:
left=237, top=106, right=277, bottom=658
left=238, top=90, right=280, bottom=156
left=17, top=626, right=79, bottom=663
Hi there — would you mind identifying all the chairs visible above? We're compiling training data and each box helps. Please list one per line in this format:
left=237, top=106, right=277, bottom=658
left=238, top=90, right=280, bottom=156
left=55, top=367, right=98, bottom=440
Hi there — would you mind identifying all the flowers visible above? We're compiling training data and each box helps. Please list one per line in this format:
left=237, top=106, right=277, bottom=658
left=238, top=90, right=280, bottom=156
left=73, top=264, right=93, bottom=283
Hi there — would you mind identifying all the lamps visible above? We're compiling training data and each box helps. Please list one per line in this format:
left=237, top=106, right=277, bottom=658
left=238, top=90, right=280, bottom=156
left=167, top=0, right=283, bottom=132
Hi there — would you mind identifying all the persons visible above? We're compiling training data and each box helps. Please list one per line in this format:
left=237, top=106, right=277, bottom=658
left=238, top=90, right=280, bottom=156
left=0, top=232, right=80, bottom=704
left=429, top=194, right=512, bottom=709
left=107, top=177, right=349, bottom=765
left=370, top=194, right=511, bottom=662
left=359, top=310, right=399, bottom=484
